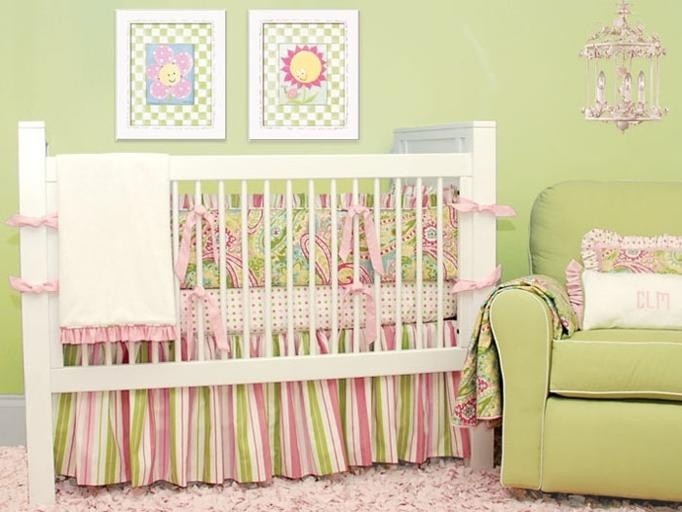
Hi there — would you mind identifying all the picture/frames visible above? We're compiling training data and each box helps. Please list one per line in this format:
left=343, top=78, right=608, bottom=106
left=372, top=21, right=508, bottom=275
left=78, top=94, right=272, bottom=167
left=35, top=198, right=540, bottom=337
left=115, top=9, right=361, bottom=142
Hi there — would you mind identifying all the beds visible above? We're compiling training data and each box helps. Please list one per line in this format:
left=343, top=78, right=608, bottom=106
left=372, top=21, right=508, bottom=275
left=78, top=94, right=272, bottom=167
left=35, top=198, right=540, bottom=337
left=16, top=119, right=498, bottom=511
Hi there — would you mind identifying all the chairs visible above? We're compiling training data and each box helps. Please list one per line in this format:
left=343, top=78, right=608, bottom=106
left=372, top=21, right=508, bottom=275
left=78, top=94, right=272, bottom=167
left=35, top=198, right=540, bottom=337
left=486, top=181, right=681, bottom=505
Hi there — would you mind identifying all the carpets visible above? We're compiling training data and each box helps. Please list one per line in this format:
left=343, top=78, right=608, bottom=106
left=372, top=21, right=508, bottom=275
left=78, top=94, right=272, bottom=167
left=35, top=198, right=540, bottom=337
left=1, top=428, right=681, bottom=512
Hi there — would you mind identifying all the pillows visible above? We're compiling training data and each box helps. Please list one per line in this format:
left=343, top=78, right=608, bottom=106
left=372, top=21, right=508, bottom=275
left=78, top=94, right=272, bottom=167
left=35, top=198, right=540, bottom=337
left=565, top=228, right=682, bottom=331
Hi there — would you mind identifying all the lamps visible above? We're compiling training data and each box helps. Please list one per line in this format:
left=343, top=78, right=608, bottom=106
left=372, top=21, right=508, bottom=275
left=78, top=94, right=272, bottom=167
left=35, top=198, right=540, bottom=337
left=578, top=7, right=670, bottom=135
